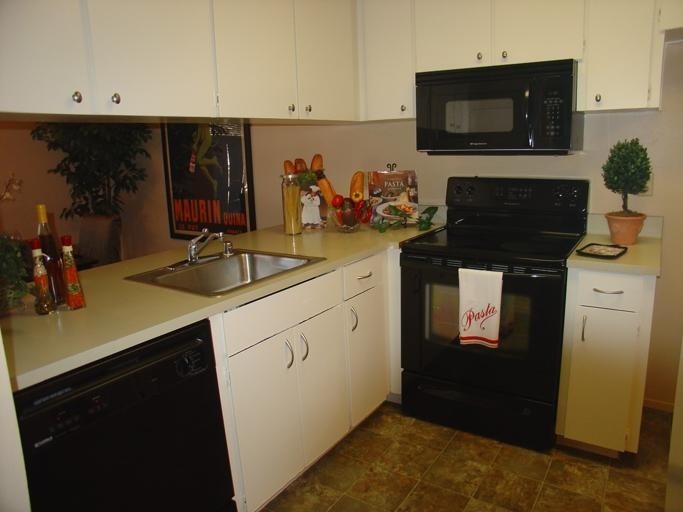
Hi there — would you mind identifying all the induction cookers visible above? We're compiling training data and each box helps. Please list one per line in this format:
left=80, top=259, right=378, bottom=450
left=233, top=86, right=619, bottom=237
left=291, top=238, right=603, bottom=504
left=400, top=213, right=584, bottom=267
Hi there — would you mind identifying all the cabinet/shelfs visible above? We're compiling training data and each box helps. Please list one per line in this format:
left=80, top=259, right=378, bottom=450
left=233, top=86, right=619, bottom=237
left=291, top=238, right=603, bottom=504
left=214, top=1, right=355, bottom=126
left=342, top=251, right=390, bottom=431
left=221, top=267, right=351, bottom=512
left=575, top=0, right=682, bottom=116
left=554, top=268, right=657, bottom=462
left=355, top=1, right=414, bottom=125
left=0, top=0, right=218, bottom=126
left=414, top=1, right=584, bottom=73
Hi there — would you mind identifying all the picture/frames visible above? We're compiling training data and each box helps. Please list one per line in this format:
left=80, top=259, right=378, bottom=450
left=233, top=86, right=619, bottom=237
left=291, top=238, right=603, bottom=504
left=158, top=118, right=256, bottom=244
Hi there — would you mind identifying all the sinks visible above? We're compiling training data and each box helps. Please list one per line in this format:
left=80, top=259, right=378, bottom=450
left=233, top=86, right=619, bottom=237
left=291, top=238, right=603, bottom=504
left=123, top=248, right=327, bottom=298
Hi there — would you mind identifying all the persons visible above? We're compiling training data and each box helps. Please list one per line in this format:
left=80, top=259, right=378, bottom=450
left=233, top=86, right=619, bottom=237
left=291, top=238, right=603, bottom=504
left=300, top=185, right=323, bottom=230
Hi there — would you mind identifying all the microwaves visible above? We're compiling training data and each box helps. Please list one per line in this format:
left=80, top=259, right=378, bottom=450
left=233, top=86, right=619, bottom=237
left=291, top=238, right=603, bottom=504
left=412, top=60, right=576, bottom=157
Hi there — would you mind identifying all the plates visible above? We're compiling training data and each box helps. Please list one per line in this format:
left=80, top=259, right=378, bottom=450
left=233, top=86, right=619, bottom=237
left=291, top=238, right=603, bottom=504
left=575, top=242, right=628, bottom=259
left=369, top=190, right=417, bottom=220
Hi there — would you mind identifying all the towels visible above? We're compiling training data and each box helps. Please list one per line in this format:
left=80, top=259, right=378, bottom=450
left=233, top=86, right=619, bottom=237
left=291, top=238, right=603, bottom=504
left=456, top=265, right=506, bottom=352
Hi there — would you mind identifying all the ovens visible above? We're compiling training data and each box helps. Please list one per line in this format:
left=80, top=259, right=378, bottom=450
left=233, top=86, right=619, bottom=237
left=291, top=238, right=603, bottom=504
left=397, top=252, right=566, bottom=452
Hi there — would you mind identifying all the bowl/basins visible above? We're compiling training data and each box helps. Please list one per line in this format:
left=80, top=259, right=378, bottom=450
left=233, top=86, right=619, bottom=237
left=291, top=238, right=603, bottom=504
left=328, top=205, right=365, bottom=233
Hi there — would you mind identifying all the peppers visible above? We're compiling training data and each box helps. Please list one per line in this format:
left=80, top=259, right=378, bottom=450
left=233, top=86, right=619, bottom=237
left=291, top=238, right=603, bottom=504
left=354, top=200, right=372, bottom=222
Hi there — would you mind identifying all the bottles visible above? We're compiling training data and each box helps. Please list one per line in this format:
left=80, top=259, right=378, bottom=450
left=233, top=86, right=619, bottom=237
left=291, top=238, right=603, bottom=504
left=27, top=239, right=56, bottom=315
left=34, top=203, right=67, bottom=305
left=280, top=173, right=302, bottom=235
left=60, top=236, right=85, bottom=309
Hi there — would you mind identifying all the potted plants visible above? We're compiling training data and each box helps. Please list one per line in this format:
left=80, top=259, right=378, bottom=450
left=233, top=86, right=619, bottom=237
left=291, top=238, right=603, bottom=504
left=29, top=122, right=154, bottom=263
left=599, top=135, right=655, bottom=247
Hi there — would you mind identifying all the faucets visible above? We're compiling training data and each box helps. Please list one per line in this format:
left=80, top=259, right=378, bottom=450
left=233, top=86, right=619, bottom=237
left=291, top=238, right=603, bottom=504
left=188, top=228, right=224, bottom=261
